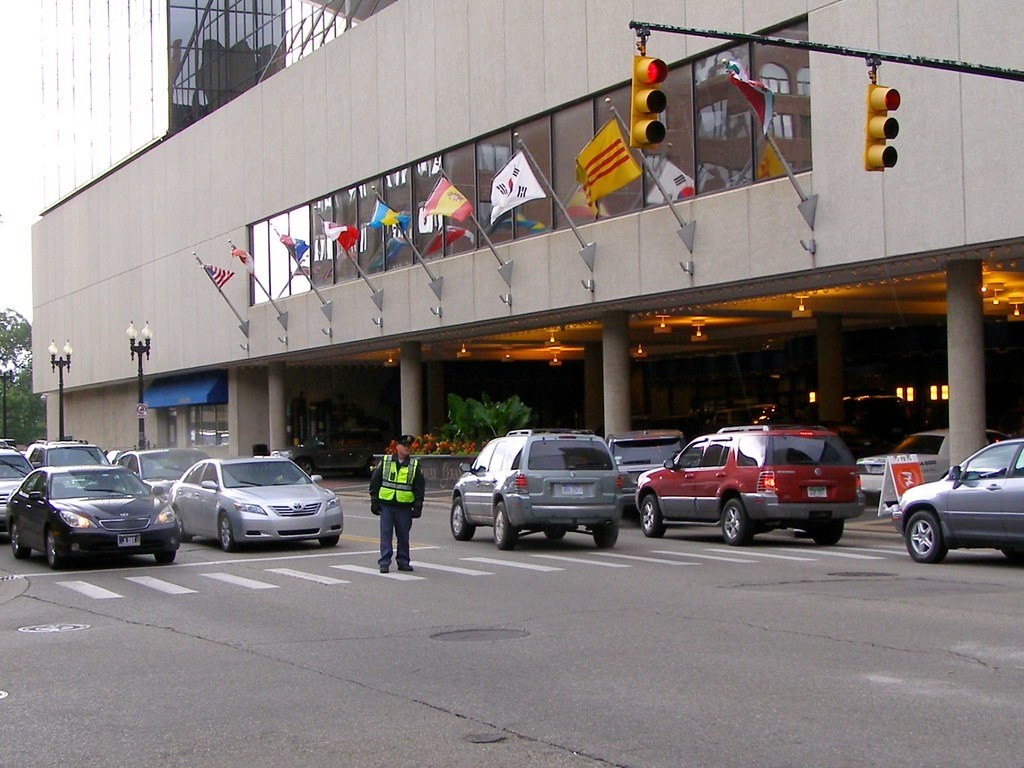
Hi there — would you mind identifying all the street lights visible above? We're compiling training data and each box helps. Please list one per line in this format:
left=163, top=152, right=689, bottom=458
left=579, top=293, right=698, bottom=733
left=48, top=338, right=73, bottom=442
left=126, top=320, right=153, bottom=451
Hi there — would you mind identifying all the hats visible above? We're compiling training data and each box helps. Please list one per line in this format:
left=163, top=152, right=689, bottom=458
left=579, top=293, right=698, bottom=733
left=394, top=435, right=415, bottom=448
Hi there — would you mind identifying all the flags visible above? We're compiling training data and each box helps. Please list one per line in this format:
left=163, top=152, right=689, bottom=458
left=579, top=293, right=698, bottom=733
left=366, top=199, right=410, bottom=232
left=231, top=247, right=254, bottom=275
left=322, top=220, right=360, bottom=254
left=722, top=57, right=774, bottom=135
left=423, top=178, right=473, bottom=223
left=200, top=263, right=235, bottom=288
left=280, top=234, right=310, bottom=261
left=295, top=131, right=789, bottom=280
left=574, top=115, right=643, bottom=203
left=489, top=150, right=547, bottom=226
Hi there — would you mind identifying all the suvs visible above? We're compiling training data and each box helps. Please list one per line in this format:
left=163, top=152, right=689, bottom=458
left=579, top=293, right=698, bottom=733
left=268, top=426, right=387, bottom=480
left=451, top=429, right=624, bottom=550
left=0, top=438, right=36, bottom=533
left=635, top=425, right=864, bottom=546
left=25, top=440, right=117, bottom=491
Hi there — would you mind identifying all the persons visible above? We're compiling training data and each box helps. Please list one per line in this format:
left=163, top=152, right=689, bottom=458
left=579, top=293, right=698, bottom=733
left=368, top=434, right=426, bottom=574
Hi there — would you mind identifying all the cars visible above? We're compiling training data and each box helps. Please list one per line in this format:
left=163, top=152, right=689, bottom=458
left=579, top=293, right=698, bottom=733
left=606, top=428, right=686, bottom=508
left=169, top=455, right=343, bottom=553
left=113, top=446, right=212, bottom=502
left=892, top=437, right=1024, bottom=565
left=855, top=428, right=1014, bottom=504
left=6, top=465, right=180, bottom=570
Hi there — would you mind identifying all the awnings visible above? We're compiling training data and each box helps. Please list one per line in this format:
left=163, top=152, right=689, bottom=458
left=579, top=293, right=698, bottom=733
left=137, top=369, right=228, bottom=408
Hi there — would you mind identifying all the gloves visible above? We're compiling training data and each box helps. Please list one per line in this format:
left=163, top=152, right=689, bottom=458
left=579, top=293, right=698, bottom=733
left=371, top=502, right=382, bottom=516
left=411, top=506, right=422, bottom=518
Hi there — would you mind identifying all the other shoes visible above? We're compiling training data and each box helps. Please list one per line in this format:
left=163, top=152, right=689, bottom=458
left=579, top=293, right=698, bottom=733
left=380, top=566, right=389, bottom=573
left=398, top=564, right=413, bottom=572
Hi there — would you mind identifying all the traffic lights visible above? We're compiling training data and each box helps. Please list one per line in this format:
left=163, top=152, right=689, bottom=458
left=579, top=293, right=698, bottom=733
left=865, top=81, right=901, bottom=172
left=629, top=54, right=669, bottom=150
